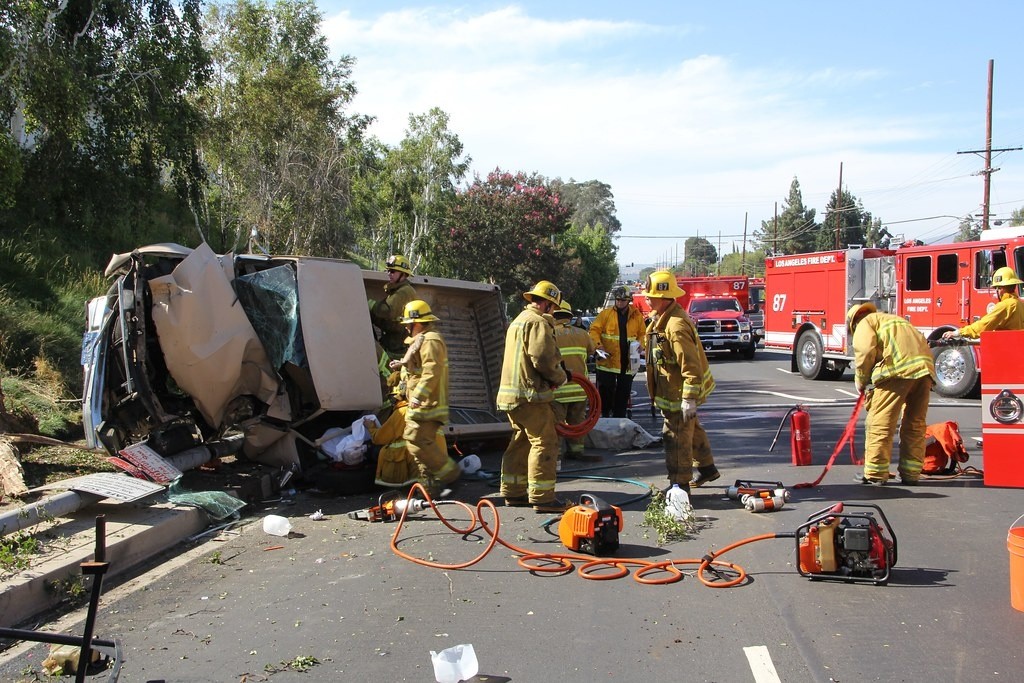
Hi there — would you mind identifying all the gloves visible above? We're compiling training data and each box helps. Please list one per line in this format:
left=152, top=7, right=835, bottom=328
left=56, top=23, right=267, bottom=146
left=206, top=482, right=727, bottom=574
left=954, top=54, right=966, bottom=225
left=855, top=382, right=864, bottom=394
left=594, top=353, right=607, bottom=361
left=681, top=398, right=697, bottom=422
left=562, top=378, right=567, bottom=386
left=638, top=355, right=646, bottom=372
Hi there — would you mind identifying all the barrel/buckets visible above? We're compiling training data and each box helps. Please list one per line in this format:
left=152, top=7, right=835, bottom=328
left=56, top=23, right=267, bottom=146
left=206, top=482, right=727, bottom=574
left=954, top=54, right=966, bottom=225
left=1007, top=514, right=1024, bottom=613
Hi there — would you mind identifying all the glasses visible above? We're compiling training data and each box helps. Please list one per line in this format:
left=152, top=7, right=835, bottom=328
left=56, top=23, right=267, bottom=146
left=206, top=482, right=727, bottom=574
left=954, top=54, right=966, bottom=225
left=388, top=269, right=402, bottom=274
left=616, top=298, right=628, bottom=301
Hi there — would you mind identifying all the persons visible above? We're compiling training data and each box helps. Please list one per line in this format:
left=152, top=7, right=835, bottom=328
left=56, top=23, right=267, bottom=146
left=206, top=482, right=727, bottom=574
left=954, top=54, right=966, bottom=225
left=367, top=254, right=418, bottom=406
left=496, top=280, right=575, bottom=512
left=551, top=298, right=595, bottom=465
left=721, top=301, right=739, bottom=311
left=645, top=271, right=721, bottom=505
left=572, top=316, right=587, bottom=331
left=363, top=300, right=464, bottom=498
left=942, top=267, right=1024, bottom=448
left=845, top=302, right=939, bottom=487
left=590, top=286, right=647, bottom=418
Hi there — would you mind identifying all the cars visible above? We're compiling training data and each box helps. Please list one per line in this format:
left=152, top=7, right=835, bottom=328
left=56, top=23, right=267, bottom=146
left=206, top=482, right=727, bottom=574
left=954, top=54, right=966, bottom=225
left=564, top=316, right=597, bottom=332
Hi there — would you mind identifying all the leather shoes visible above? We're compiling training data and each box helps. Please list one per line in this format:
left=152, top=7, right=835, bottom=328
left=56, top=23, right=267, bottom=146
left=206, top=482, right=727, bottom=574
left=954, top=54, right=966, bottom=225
left=896, top=473, right=918, bottom=485
left=854, top=473, right=885, bottom=486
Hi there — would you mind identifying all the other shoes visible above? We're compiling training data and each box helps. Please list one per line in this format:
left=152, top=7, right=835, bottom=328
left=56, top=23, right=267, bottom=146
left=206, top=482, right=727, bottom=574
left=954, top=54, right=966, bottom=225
left=533, top=497, right=580, bottom=511
left=504, top=495, right=534, bottom=506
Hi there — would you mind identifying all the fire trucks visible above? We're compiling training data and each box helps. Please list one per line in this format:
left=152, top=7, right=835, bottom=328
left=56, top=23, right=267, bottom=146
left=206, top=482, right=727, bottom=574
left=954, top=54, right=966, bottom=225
left=763, top=225, right=1024, bottom=399
left=672, top=275, right=755, bottom=361
left=743, top=278, right=766, bottom=345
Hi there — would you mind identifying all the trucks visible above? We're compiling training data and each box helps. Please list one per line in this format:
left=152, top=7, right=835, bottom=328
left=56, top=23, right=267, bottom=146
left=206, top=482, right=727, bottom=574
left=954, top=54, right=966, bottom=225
left=80, top=241, right=516, bottom=482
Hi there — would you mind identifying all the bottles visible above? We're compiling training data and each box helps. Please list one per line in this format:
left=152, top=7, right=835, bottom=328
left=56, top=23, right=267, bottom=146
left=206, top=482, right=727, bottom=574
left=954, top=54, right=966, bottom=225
left=280, top=489, right=301, bottom=496
left=665, top=484, right=690, bottom=521
left=263, top=515, right=293, bottom=536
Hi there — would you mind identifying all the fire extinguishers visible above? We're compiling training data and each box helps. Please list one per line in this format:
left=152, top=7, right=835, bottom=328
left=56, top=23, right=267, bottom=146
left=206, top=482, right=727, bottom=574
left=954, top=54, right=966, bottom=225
left=769, top=402, right=812, bottom=466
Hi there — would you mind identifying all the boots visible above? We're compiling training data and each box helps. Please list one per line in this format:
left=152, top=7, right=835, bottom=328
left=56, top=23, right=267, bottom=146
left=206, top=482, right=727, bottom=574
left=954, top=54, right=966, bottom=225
left=689, top=463, right=720, bottom=487
left=659, top=483, right=691, bottom=496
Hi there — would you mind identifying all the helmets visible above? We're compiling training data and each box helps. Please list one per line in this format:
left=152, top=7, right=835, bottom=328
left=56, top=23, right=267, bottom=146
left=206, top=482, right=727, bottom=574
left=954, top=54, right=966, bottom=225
left=847, top=302, right=876, bottom=335
left=523, top=280, right=561, bottom=307
left=389, top=381, right=406, bottom=395
left=385, top=255, right=416, bottom=277
left=614, top=285, right=632, bottom=300
left=990, top=266, right=1024, bottom=287
left=553, top=300, right=574, bottom=316
left=641, top=271, right=686, bottom=298
left=400, top=300, right=440, bottom=323
